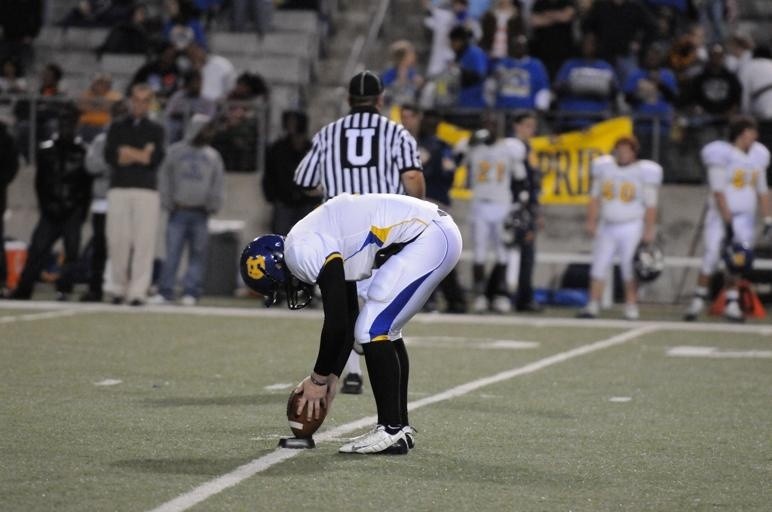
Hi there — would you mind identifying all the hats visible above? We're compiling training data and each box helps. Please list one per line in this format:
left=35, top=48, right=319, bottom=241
left=350, top=70, right=384, bottom=96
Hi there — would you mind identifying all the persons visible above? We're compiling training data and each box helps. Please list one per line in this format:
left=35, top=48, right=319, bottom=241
left=0, top=0, right=772, bottom=323
left=239, top=193, right=464, bottom=455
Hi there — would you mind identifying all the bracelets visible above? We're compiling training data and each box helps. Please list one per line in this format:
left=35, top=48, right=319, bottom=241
left=310, top=375, right=327, bottom=386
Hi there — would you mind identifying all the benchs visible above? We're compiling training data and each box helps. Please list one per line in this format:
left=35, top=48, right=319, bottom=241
left=0, top=0, right=330, bottom=166
left=206, top=220, right=245, bottom=300
left=452, top=246, right=772, bottom=316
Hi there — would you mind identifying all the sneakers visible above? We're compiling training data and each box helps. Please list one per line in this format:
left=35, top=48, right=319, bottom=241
left=340, top=374, right=364, bottom=393
left=339, top=425, right=419, bottom=455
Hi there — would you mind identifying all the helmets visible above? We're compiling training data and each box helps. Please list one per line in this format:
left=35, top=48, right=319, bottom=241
left=240, top=234, right=312, bottom=310
left=497, top=206, right=534, bottom=248
left=722, top=240, right=756, bottom=275
left=635, top=244, right=665, bottom=281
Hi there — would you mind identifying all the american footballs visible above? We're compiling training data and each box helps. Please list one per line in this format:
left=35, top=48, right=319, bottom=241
left=287, top=386, right=327, bottom=438
